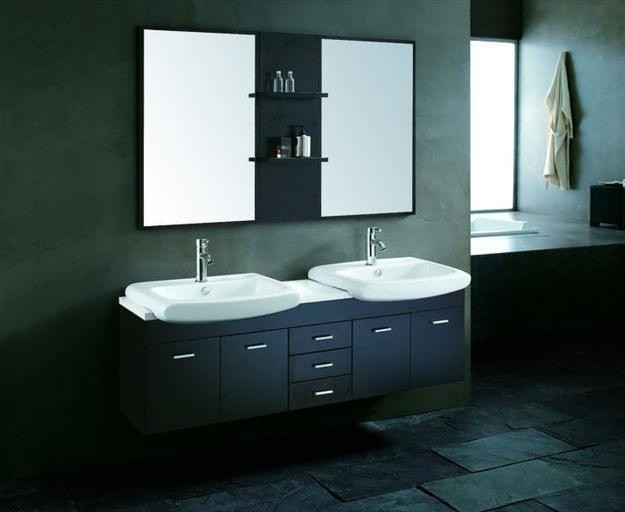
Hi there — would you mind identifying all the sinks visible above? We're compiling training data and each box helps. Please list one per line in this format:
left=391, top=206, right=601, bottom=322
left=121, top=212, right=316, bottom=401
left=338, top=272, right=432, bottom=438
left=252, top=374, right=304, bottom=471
left=308, top=256, right=471, bottom=303
left=125, top=273, right=300, bottom=325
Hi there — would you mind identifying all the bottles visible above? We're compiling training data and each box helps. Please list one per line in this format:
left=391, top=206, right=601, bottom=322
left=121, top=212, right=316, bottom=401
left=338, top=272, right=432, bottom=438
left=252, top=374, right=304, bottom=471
left=285, top=71, right=295, bottom=93
left=274, top=70, right=283, bottom=92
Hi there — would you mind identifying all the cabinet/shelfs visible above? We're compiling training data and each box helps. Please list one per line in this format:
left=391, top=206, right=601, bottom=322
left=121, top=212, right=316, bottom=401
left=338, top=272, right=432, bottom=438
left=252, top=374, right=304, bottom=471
left=248, top=88, right=328, bottom=165
left=118, top=308, right=289, bottom=436
left=354, top=290, right=466, bottom=400
left=289, top=299, right=354, bottom=415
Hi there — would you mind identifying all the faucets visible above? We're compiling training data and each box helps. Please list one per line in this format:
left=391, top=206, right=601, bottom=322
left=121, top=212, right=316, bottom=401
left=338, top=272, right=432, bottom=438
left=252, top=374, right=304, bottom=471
left=367, top=226, right=386, bottom=265
left=196, top=238, right=213, bottom=282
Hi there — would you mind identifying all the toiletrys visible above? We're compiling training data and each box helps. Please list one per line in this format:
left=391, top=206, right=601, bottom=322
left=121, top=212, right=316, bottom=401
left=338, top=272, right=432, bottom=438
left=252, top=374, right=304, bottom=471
left=295, top=125, right=311, bottom=158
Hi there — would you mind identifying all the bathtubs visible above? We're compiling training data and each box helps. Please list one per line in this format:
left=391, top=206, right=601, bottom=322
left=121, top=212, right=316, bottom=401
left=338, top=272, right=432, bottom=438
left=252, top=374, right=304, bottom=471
left=470, top=216, right=538, bottom=237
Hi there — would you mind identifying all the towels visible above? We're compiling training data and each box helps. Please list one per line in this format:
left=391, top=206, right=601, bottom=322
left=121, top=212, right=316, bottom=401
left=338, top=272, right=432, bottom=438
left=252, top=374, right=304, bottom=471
left=541, top=50, right=575, bottom=193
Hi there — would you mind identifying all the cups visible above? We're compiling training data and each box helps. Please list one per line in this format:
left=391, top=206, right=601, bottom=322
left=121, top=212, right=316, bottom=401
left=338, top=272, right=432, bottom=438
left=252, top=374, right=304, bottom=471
left=280, top=137, right=291, bottom=159
